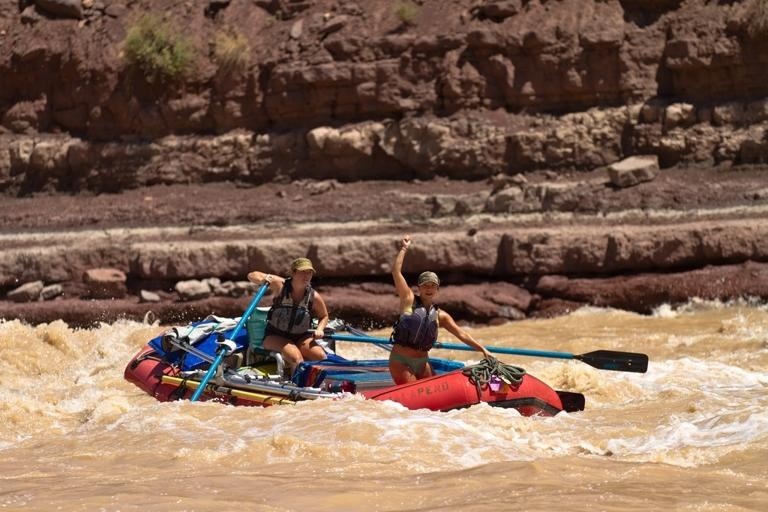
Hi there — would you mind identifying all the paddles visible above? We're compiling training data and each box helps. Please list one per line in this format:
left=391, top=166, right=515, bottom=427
left=312, top=332, right=649, bottom=373
left=556, top=390, right=585, bottom=412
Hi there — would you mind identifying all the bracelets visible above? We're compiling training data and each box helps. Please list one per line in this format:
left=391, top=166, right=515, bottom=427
left=401, top=246, right=408, bottom=252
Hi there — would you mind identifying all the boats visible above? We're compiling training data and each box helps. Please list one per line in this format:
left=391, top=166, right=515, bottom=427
left=124, top=316, right=564, bottom=420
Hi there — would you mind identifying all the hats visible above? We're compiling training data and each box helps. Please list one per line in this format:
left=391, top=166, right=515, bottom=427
left=290, top=257, right=316, bottom=274
left=418, top=271, right=440, bottom=287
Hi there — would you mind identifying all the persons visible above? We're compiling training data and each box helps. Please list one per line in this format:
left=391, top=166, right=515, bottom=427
left=388, top=234, right=496, bottom=387
left=247, top=257, right=328, bottom=381
